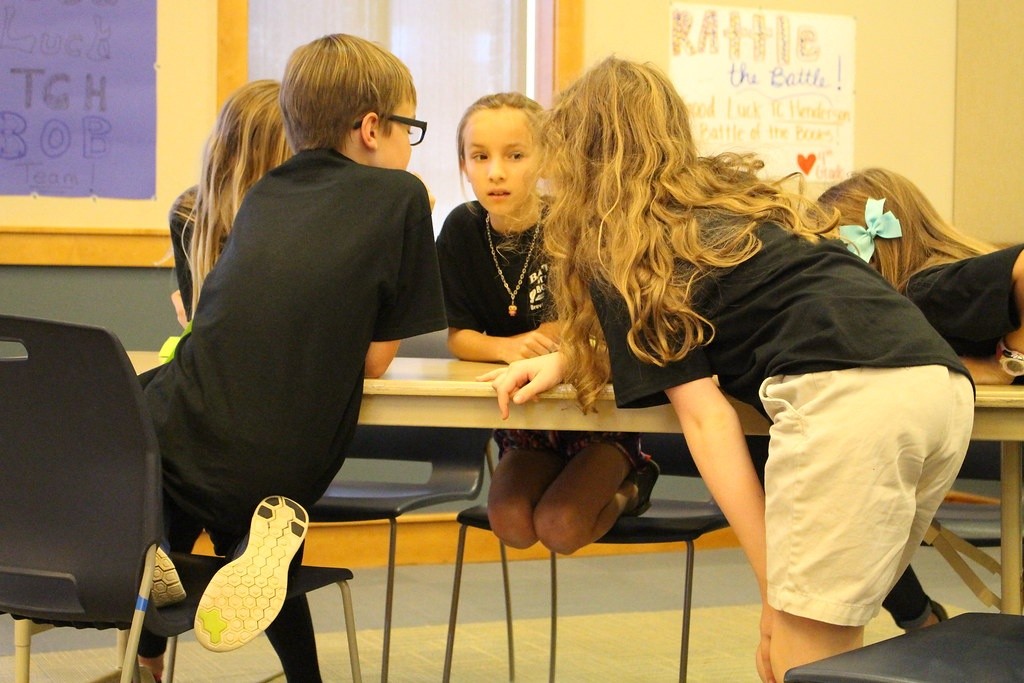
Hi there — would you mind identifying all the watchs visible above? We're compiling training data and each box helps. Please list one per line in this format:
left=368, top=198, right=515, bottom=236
left=995, top=337, right=1024, bottom=376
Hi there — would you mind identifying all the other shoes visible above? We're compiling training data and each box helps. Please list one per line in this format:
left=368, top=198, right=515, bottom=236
left=193, top=495, right=310, bottom=653
left=622, top=456, right=661, bottom=518
left=139, top=665, right=161, bottom=683
left=149, top=548, right=186, bottom=609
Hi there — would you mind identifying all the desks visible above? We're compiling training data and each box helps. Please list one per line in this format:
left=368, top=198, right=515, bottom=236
left=115, top=347, right=1024, bottom=626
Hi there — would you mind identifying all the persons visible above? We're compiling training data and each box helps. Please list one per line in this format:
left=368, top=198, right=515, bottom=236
left=436, top=92, right=660, bottom=556
left=476, top=57, right=1024, bottom=683
left=135, top=33, right=449, bottom=683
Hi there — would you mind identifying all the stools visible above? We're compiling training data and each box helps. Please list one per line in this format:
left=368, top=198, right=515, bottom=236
left=783, top=612, right=1024, bottom=683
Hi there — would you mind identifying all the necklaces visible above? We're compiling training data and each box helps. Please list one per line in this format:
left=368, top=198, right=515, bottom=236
left=485, top=213, right=542, bottom=317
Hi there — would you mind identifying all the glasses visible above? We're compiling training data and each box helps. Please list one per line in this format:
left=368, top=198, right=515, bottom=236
left=351, top=113, right=428, bottom=146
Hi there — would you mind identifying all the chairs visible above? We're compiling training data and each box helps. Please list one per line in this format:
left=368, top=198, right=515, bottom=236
left=1, top=314, right=362, bottom=683
left=442, top=449, right=733, bottom=683
left=304, top=319, right=519, bottom=683
